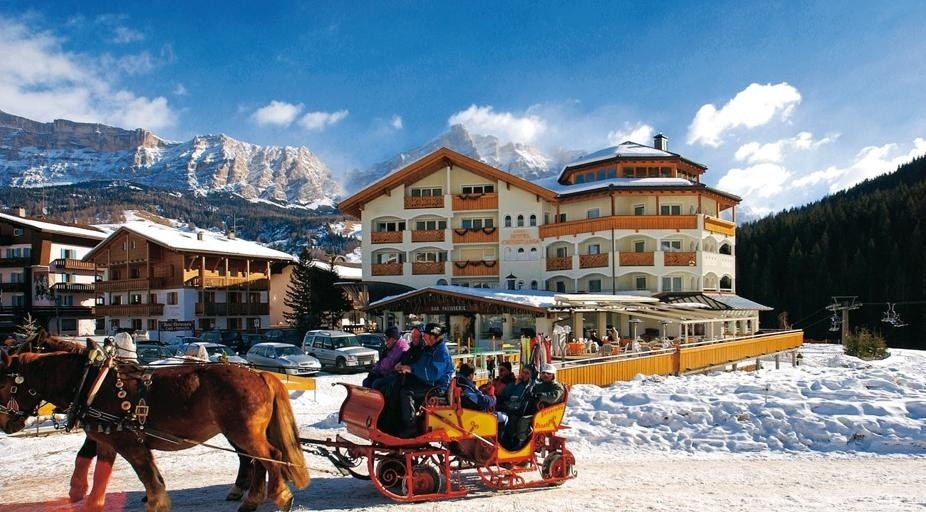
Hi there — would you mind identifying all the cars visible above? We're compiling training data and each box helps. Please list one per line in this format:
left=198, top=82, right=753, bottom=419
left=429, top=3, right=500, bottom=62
left=246, top=340, right=323, bottom=375
left=113, top=327, right=304, bottom=370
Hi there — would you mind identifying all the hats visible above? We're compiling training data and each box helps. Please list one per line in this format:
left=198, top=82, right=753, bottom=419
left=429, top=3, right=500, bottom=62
left=419, top=323, right=447, bottom=335
left=499, top=361, right=512, bottom=371
left=413, top=323, right=425, bottom=332
left=542, top=364, right=556, bottom=374
left=384, top=326, right=400, bottom=338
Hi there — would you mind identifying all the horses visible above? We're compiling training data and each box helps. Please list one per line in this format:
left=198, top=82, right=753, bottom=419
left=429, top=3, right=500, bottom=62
left=0, top=348, right=310, bottom=512
left=13, top=326, right=288, bottom=503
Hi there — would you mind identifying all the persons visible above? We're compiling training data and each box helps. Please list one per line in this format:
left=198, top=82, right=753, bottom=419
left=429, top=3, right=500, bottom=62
left=233, top=332, right=244, bottom=356
left=590, top=330, right=603, bottom=346
left=363, top=322, right=565, bottom=451
left=608, top=327, right=618, bottom=341
left=568, top=332, right=576, bottom=342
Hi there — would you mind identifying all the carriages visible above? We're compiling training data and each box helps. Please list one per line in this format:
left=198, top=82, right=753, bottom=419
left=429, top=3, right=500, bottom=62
left=0, top=328, right=578, bottom=511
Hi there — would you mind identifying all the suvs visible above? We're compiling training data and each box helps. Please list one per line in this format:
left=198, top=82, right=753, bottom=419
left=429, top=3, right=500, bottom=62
left=301, top=329, right=380, bottom=373
left=355, top=332, right=390, bottom=359
